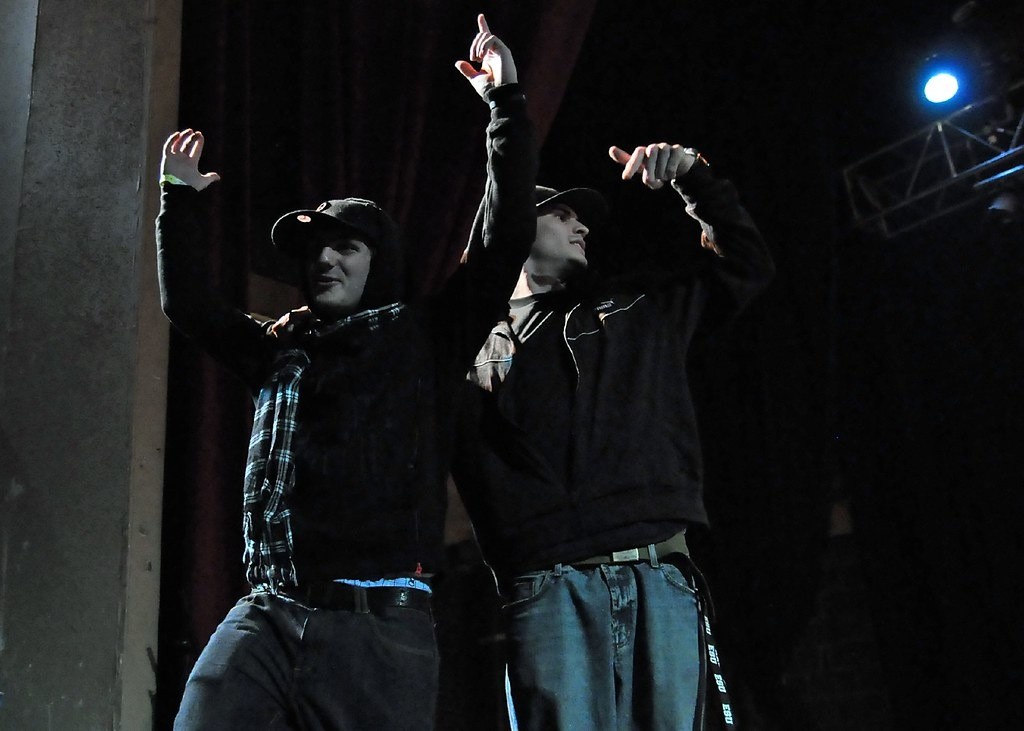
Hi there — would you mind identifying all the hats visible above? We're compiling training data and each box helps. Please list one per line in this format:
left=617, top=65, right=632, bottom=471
left=534, top=183, right=609, bottom=238
left=270, top=197, right=421, bottom=293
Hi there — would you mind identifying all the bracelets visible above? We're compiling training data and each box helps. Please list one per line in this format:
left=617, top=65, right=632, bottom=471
left=159, top=174, right=187, bottom=187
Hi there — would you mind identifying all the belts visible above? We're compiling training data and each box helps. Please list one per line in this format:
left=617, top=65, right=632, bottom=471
left=250, top=579, right=433, bottom=614
left=574, top=533, right=716, bottom=627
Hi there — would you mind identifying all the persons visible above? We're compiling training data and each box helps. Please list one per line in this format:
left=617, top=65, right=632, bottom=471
left=450, top=143, right=775, bottom=731
left=155, top=13, right=537, bottom=731
